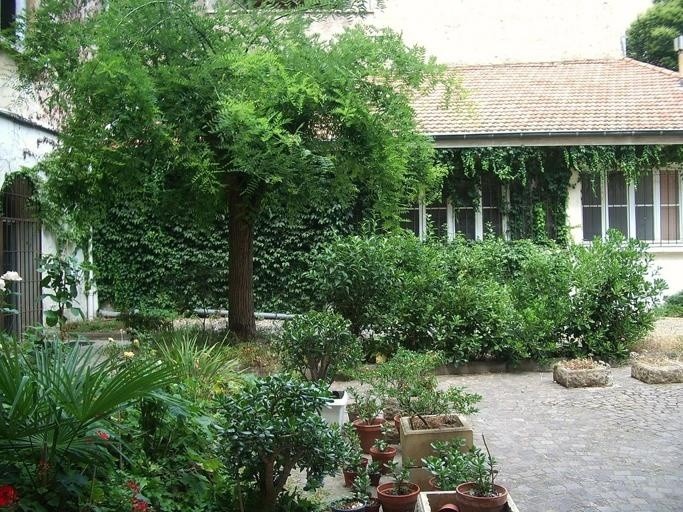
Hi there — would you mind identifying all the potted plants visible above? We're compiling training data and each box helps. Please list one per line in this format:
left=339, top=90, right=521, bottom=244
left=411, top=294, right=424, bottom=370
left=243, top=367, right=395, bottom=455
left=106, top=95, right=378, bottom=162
left=269, top=309, right=508, bottom=510
